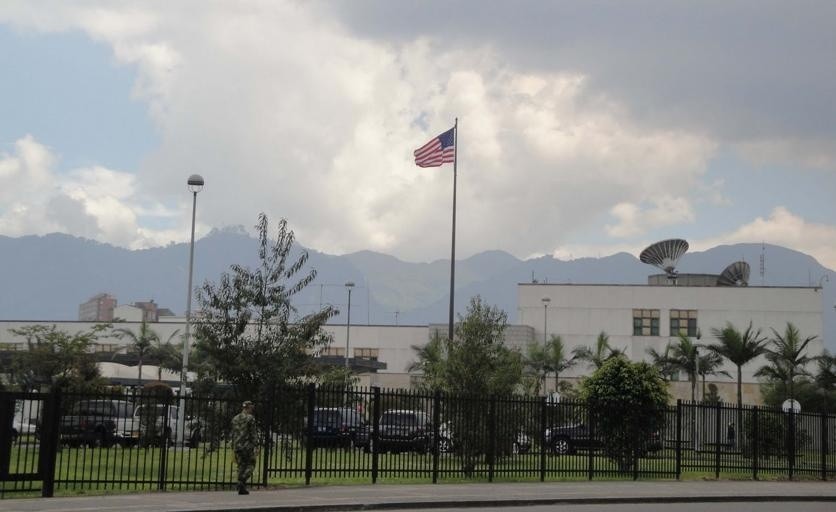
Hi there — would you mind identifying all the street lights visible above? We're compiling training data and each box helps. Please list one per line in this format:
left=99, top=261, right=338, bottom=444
left=343, top=279, right=356, bottom=411
left=176, top=174, right=204, bottom=447
left=541, top=295, right=551, bottom=394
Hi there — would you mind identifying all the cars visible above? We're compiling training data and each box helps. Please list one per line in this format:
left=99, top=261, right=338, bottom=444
left=545, top=415, right=662, bottom=454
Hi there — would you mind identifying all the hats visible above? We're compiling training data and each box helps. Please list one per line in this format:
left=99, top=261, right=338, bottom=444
left=241, top=400, right=256, bottom=409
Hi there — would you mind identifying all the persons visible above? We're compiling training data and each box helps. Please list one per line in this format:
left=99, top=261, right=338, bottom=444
left=230, top=398, right=263, bottom=495
left=725, top=421, right=738, bottom=452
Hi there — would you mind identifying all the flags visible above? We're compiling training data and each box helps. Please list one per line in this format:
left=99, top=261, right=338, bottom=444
left=413, top=124, right=454, bottom=170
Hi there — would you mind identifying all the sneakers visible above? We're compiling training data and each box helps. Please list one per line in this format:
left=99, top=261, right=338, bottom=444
left=235, top=480, right=250, bottom=496
